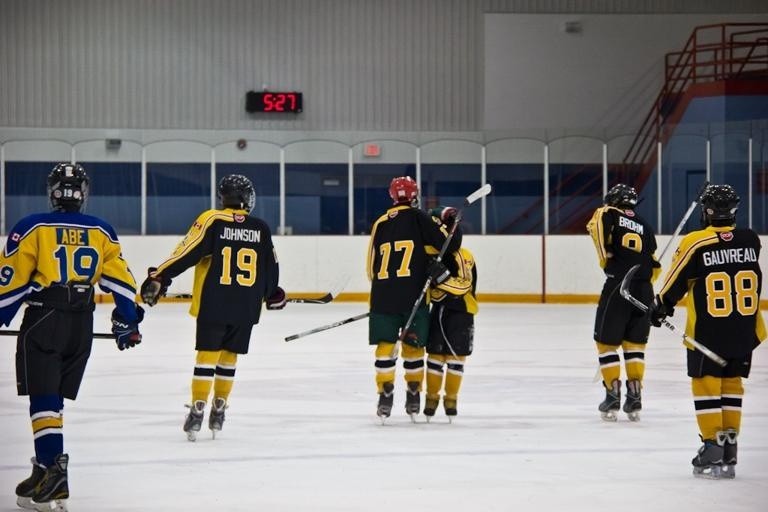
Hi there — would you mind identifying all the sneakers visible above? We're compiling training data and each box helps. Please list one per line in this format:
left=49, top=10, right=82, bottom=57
left=725, top=429, right=737, bottom=463
left=622, top=380, right=643, bottom=412
left=424, top=394, right=440, bottom=415
left=377, top=393, right=393, bottom=417
left=15, top=457, right=57, bottom=497
left=599, top=379, right=620, bottom=412
left=32, top=455, right=69, bottom=502
left=405, top=391, right=420, bottom=413
left=444, top=395, right=457, bottom=413
left=183, top=401, right=204, bottom=433
left=206, top=397, right=226, bottom=430
left=693, top=432, right=726, bottom=468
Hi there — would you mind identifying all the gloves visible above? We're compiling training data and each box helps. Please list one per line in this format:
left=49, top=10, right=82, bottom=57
left=141, top=268, right=168, bottom=304
left=606, top=260, right=622, bottom=275
left=267, top=286, right=286, bottom=309
left=111, top=311, right=144, bottom=350
left=645, top=300, right=665, bottom=328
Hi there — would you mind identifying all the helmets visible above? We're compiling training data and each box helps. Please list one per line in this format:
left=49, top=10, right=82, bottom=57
left=49, top=163, right=88, bottom=212
left=217, top=173, right=257, bottom=213
left=702, top=184, right=739, bottom=220
left=391, top=178, right=418, bottom=204
left=607, top=185, right=637, bottom=210
left=428, top=204, right=460, bottom=228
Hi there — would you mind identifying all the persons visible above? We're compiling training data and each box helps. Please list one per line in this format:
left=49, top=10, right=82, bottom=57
left=645, top=183, right=762, bottom=480
left=140, top=173, right=286, bottom=443
left=365, top=175, right=450, bottom=424
left=0, top=163, right=145, bottom=512
left=587, top=181, right=661, bottom=424
left=422, top=204, right=477, bottom=422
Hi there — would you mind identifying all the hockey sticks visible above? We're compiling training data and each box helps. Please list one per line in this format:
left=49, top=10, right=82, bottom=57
left=160, top=272, right=351, bottom=305
left=619, top=263, right=727, bottom=367
left=390, top=184, right=491, bottom=358
